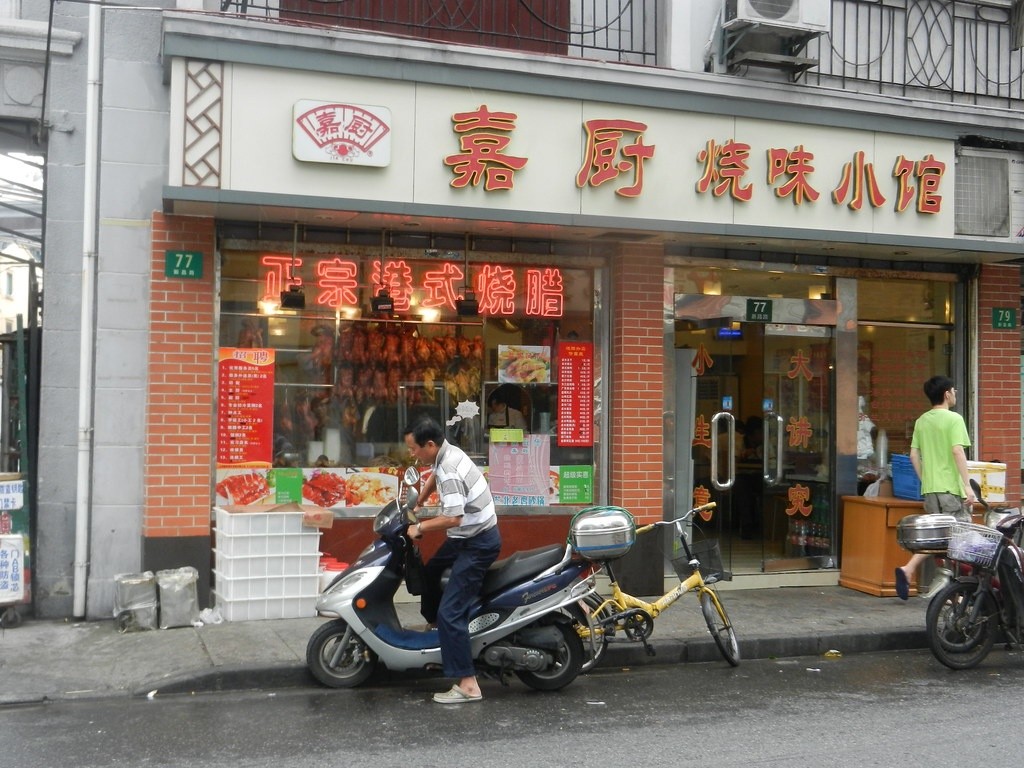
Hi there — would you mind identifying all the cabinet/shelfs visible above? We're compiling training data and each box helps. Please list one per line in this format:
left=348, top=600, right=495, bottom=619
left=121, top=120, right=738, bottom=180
left=838, top=495, right=1009, bottom=598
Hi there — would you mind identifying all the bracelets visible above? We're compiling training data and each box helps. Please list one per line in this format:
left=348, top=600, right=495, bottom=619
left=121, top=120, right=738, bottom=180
left=417, top=523, right=423, bottom=533
left=417, top=502, right=424, bottom=507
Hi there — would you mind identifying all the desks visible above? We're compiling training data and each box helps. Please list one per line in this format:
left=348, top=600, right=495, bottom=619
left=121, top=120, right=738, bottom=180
left=736, top=461, right=793, bottom=471
left=786, top=474, right=830, bottom=484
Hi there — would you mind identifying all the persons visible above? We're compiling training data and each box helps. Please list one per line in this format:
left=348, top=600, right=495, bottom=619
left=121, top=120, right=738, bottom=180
left=487, top=396, right=529, bottom=431
left=895, top=375, right=974, bottom=606
left=404, top=415, right=502, bottom=703
left=705, top=415, right=796, bottom=540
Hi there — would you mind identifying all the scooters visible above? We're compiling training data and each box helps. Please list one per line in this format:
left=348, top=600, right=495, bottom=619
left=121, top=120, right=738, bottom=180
left=306, top=466, right=637, bottom=693
left=897, top=480, right=1024, bottom=671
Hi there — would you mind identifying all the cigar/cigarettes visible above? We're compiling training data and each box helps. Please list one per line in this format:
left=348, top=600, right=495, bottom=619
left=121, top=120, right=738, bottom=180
left=414, top=459, right=418, bottom=466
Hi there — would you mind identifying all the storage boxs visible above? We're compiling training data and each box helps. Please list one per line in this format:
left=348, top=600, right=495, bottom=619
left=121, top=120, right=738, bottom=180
left=211, top=507, right=323, bottom=622
left=890, top=453, right=927, bottom=501
left=963, top=461, right=1007, bottom=503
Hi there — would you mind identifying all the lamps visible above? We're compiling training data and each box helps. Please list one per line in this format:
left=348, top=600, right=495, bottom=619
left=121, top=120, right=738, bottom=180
left=281, top=278, right=306, bottom=309
left=370, top=281, right=395, bottom=314
left=456, top=286, right=478, bottom=315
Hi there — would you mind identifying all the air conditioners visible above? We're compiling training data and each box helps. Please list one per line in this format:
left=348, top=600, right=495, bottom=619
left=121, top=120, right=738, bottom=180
left=720, top=0, right=830, bottom=38
left=696, top=375, right=739, bottom=437
left=955, top=146, right=1024, bottom=244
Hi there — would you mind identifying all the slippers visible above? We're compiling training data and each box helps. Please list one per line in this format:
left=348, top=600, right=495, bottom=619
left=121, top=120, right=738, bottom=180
left=432, top=685, right=483, bottom=703
left=895, top=567, right=910, bottom=600
left=946, top=595, right=974, bottom=606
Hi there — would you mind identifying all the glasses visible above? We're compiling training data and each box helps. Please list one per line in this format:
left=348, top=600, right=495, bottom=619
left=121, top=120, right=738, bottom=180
left=951, top=389, right=958, bottom=394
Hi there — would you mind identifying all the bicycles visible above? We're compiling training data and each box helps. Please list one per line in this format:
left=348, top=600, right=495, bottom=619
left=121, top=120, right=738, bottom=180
left=556, top=499, right=741, bottom=673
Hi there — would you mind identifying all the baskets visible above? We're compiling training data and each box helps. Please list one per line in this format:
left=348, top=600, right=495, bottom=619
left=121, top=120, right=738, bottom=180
left=947, top=521, right=1003, bottom=567
left=669, top=538, right=724, bottom=587
